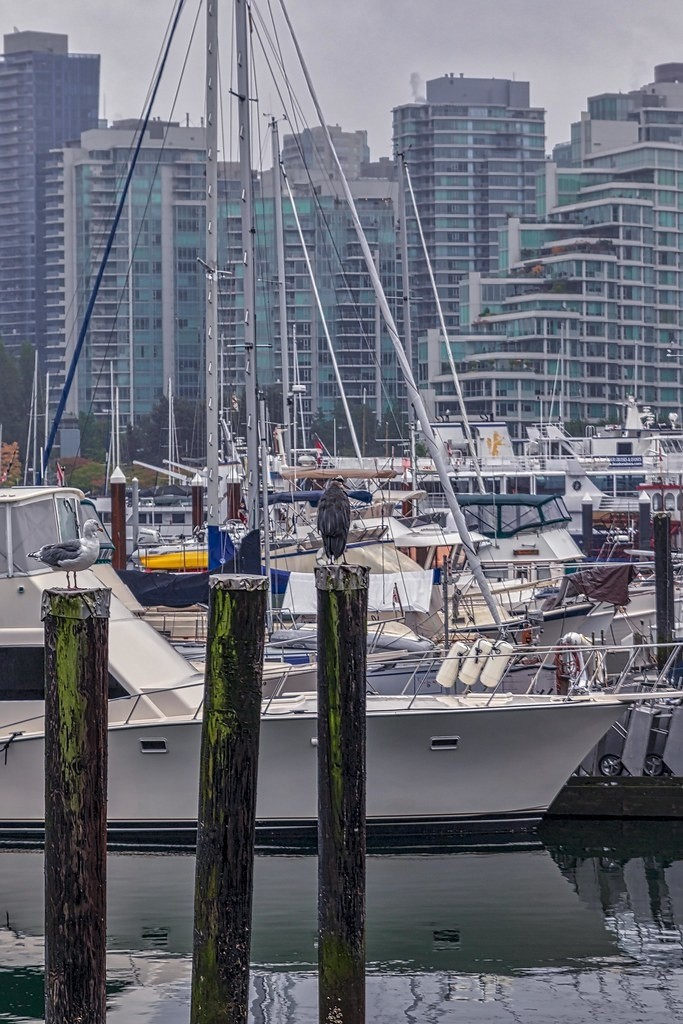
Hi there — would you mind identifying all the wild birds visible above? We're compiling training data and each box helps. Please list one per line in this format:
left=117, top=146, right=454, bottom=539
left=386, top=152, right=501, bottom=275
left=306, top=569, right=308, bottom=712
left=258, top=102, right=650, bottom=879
left=315, top=476, right=352, bottom=565
left=26, top=518, right=104, bottom=589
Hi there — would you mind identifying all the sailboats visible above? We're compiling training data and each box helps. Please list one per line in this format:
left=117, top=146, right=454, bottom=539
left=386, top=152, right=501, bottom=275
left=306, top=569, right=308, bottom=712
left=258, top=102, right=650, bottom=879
left=2, top=825, right=640, bottom=1023
left=0, top=1, right=682, bottom=827
